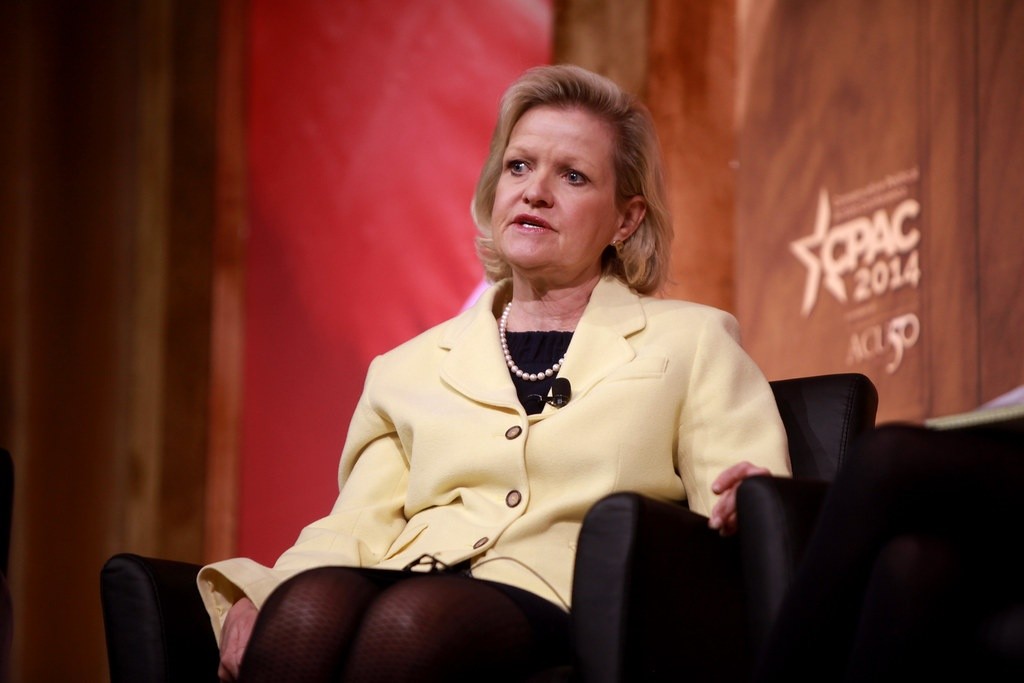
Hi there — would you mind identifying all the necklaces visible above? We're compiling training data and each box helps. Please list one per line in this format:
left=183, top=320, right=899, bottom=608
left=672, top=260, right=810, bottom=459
left=500, top=299, right=565, bottom=380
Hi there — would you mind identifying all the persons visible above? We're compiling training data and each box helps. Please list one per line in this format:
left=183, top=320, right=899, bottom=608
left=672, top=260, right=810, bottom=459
left=197, top=64, right=790, bottom=683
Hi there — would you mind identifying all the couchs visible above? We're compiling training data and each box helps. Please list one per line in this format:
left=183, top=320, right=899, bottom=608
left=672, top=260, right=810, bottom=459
left=97, top=371, right=1024, bottom=683
